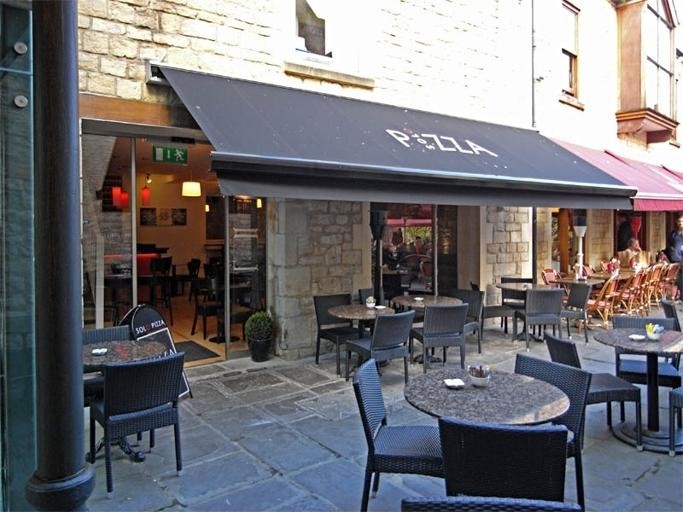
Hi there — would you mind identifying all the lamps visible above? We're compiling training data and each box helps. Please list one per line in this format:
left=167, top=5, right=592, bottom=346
left=181, top=161, right=201, bottom=197
left=204, top=196, right=210, bottom=213
left=111, top=155, right=129, bottom=210
left=573, top=225, right=589, bottom=279
left=141, top=172, right=152, bottom=207
left=146, top=173, right=152, bottom=184
left=256, top=197, right=262, bottom=209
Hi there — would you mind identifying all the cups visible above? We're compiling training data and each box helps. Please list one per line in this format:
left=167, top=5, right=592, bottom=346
left=647, top=332, right=661, bottom=340
left=468, top=373, right=489, bottom=387
left=413, top=298, right=424, bottom=304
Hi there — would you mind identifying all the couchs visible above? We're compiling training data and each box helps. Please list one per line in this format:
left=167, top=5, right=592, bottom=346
left=104, top=253, right=162, bottom=305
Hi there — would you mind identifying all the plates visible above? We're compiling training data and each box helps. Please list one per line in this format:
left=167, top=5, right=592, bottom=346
left=375, top=306, right=386, bottom=310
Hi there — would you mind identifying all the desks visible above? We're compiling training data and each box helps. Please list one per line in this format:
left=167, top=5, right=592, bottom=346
left=393, top=294, right=463, bottom=363
left=406, top=368, right=572, bottom=425
left=329, top=304, right=395, bottom=369
left=82, top=341, right=167, bottom=407
left=105, top=274, right=153, bottom=326
left=592, top=326, right=683, bottom=454
left=495, top=283, right=551, bottom=342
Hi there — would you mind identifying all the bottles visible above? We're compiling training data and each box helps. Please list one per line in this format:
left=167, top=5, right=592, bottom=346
left=660, top=251, right=664, bottom=262
left=608, top=264, right=614, bottom=273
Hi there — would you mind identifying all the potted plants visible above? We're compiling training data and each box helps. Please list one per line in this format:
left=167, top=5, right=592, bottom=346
left=244, top=313, right=275, bottom=362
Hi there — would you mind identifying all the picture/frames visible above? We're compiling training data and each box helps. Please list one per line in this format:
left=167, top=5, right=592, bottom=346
left=156, top=208, right=172, bottom=226
left=140, top=208, right=156, bottom=225
left=172, top=208, right=187, bottom=226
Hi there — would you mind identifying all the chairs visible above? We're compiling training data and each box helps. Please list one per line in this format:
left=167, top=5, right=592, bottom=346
left=515, top=353, right=592, bottom=511
left=345, top=310, right=416, bottom=386
left=191, top=285, right=222, bottom=339
left=401, top=493, right=581, bottom=511
left=612, top=315, right=675, bottom=422
left=397, top=253, right=421, bottom=281
left=513, top=289, right=564, bottom=352
left=420, top=258, right=433, bottom=290
left=82, top=325, right=129, bottom=344
left=140, top=256, right=172, bottom=309
left=450, top=290, right=484, bottom=354
left=188, top=258, right=213, bottom=301
left=401, top=418, right=568, bottom=500
left=500, top=277, right=533, bottom=328
left=553, top=283, right=592, bottom=346
left=87, top=352, right=184, bottom=499
left=540, top=250, right=683, bottom=334
left=409, top=303, right=469, bottom=373
left=358, top=288, right=376, bottom=338
left=668, top=386, right=683, bottom=457
left=542, top=330, right=644, bottom=454
left=314, top=294, right=369, bottom=377
left=352, top=359, right=447, bottom=512
left=661, top=296, right=682, bottom=332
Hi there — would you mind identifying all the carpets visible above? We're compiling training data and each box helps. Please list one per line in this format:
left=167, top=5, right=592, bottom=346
left=174, top=341, right=220, bottom=363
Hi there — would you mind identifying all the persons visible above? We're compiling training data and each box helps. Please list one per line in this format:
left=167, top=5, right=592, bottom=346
left=617, top=237, right=642, bottom=270
left=667, top=211, right=683, bottom=301
